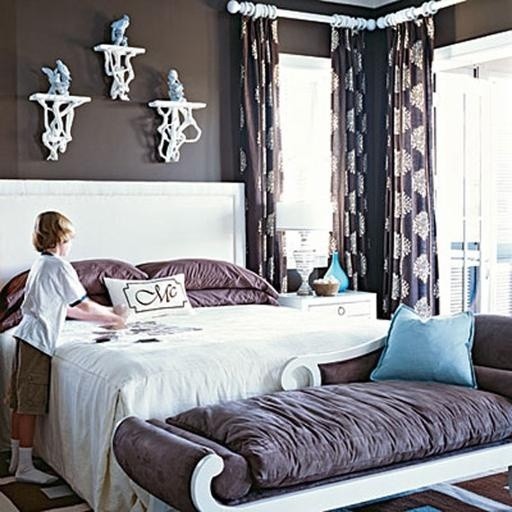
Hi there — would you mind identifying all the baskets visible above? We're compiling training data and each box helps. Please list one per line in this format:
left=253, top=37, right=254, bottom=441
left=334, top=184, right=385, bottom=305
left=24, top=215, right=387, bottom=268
left=312, top=277, right=340, bottom=297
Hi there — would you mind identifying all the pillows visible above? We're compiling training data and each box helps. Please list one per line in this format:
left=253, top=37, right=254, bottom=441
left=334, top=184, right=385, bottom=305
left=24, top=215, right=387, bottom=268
left=1, top=259, right=281, bottom=332
left=368, top=302, right=478, bottom=389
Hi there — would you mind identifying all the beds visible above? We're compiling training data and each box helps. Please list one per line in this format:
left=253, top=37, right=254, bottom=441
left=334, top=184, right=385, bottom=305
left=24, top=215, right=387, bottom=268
left=0, top=179, right=391, bottom=512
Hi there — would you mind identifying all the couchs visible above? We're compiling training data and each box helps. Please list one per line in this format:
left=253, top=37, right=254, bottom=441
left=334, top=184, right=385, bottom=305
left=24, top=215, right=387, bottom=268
left=110, top=314, right=511, bottom=512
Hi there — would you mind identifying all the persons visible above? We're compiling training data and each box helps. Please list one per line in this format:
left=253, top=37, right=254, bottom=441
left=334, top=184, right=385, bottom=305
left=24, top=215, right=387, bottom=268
left=8, top=211, right=126, bottom=485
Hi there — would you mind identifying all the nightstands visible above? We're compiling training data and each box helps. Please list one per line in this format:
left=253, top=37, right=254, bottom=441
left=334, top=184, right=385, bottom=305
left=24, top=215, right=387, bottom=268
left=279, top=291, right=378, bottom=323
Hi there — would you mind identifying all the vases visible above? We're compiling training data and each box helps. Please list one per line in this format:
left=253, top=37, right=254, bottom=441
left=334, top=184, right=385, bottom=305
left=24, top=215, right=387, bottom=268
left=318, top=254, right=349, bottom=291
